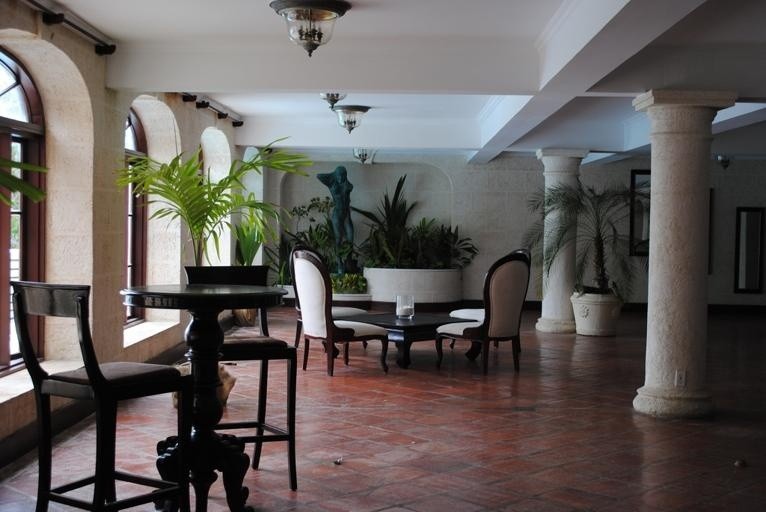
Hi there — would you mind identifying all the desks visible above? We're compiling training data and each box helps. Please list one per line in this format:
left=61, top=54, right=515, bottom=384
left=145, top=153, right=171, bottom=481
left=113, top=282, right=291, bottom=511
left=330, top=310, right=476, bottom=368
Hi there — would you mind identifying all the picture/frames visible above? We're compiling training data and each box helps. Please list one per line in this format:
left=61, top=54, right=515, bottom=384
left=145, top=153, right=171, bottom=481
left=732, top=206, right=766, bottom=296
left=629, top=167, right=652, bottom=258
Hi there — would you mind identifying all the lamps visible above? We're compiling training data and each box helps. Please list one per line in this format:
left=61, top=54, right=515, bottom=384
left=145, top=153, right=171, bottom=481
left=267, top=0, right=353, bottom=60
left=315, top=91, right=347, bottom=109
left=328, top=104, right=373, bottom=136
left=352, top=147, right=374, bottom=166
left=714, top=153, right=737, bottom=171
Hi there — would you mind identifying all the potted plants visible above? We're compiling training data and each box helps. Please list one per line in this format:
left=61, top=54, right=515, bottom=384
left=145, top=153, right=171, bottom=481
left=225, top=191, right=272, bottom=327
left=349, top=171, right=480, bottom=314
left=518, top=176, right=657, bottom=339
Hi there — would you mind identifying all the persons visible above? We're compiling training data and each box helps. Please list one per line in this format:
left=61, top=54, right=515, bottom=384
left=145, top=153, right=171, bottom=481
left=316, top=165, right=355, bottom=273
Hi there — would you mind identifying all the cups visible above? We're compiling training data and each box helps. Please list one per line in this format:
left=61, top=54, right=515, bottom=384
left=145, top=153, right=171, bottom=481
left=394, top=293, right=416, bottom=321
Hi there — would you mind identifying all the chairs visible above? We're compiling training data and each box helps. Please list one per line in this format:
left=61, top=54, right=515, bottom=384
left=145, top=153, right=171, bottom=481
left=6, top=278, right=191, bottom=511
left=283, top=244, right=392, bottom=377
left=175, top=264, right=301, bottom=493
left=432, top=247, right=532, bottom=374
